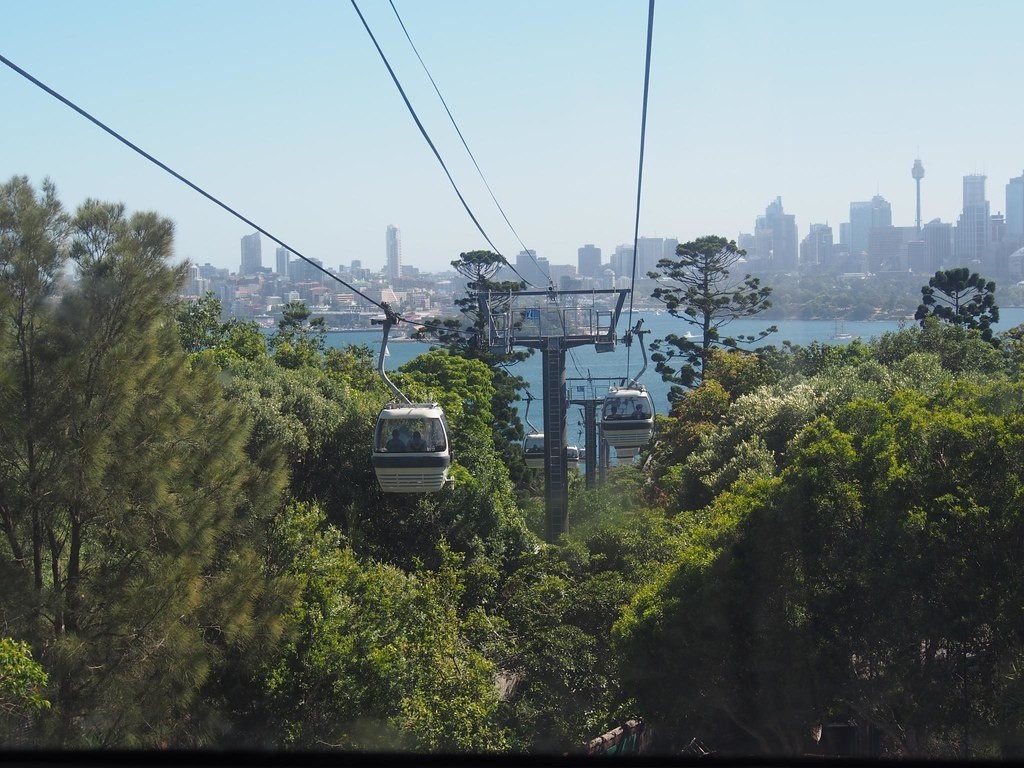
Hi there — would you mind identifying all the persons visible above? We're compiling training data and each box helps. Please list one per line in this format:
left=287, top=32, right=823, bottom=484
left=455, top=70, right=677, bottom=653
left=527, top=444, right=537, bottom=453
left=385, top=429, right=427, bottom=451
left=607, top=406, right=622, bottom=417
left=632, top=404, right=646, bottom=416
